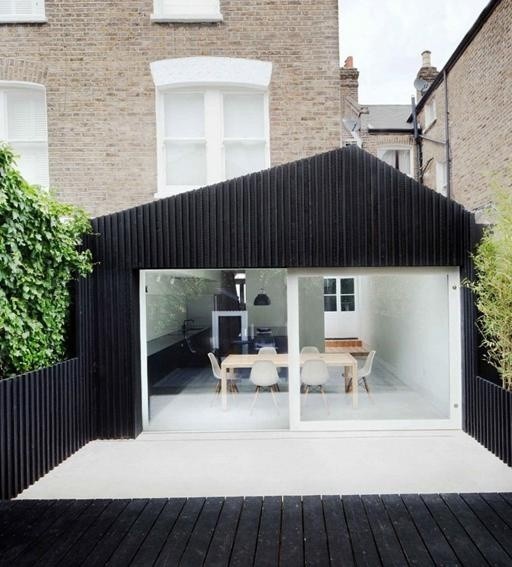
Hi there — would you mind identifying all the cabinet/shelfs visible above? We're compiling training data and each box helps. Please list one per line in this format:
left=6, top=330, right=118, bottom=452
left=149, top=326, right=208, bottom=386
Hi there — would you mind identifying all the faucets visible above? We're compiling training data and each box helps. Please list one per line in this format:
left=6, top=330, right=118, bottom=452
left=184, top=319, right=193, bottom=326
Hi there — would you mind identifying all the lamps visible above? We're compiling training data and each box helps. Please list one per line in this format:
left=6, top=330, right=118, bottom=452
left=252, top=287, right=273, bottom=306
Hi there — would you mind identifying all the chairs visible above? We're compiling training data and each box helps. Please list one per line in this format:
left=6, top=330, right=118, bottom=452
left=206, top=341, right=377, bottom=411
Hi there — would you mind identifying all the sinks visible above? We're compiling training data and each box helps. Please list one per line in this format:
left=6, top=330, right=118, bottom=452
left=188, top=327, right=204, bottom=330
